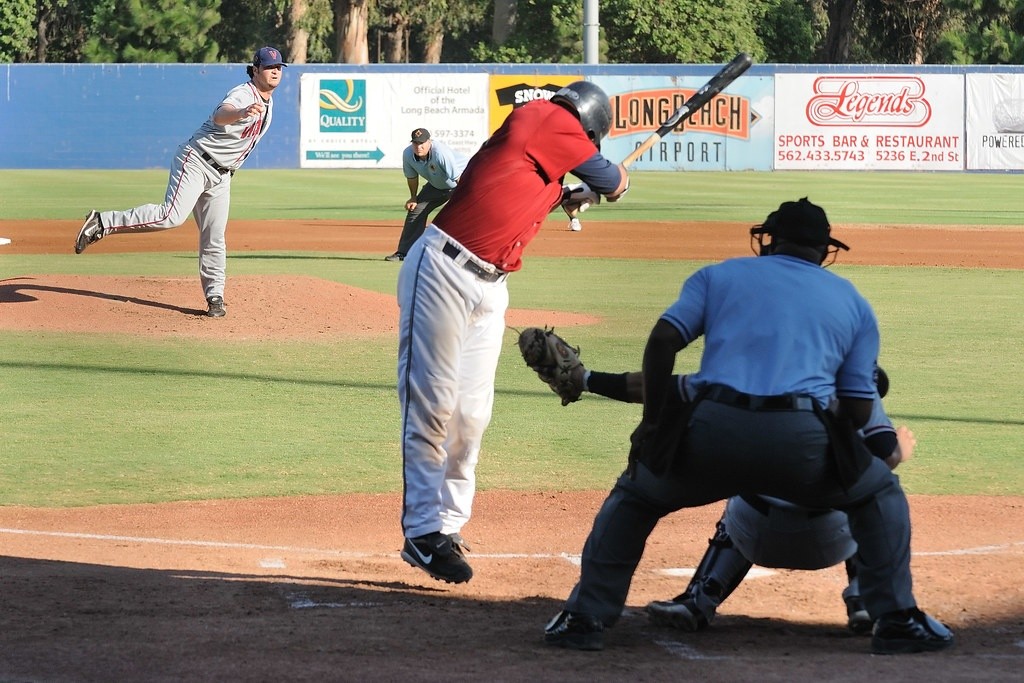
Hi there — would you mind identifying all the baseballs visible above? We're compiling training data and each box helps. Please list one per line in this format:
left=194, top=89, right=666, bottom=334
left=561, top=52, right=756, bottom=220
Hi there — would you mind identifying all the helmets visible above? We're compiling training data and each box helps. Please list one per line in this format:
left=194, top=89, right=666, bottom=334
left=551, top=81, right=614, bottom=154
left=758, top=196, right=831, bottom=264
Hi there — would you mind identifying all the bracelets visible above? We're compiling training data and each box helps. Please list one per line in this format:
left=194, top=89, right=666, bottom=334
left=241, top=108, right=247, bottom=119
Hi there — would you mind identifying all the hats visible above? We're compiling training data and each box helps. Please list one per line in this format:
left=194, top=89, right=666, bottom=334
left=411, top=128, right=430, bottom=142
left=253, top=46, right=287, bottom=67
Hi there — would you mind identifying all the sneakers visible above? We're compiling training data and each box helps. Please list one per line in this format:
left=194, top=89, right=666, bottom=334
left=647, top=598, right=709, bottom=632
left=871, top=617, right=952, bottom=652
left=569, top=218, right=581, bottom=230
left=385, top=251, right=406, bottom=261
left=545, top=612, right=605, bottom=650
left=401, top=532, right=473, bottom=582
left=206, top=296, right=226, bottom=316
left=75, top=210, right=104, bottom=254
left=846, top=616, right=872, bottom=636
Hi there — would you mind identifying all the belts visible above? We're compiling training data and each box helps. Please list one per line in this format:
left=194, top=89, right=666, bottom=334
left=741, top=495, right=833, bottom=524
left=189, top=136, right=229, bottom=175
left=695, top=383, right=814, bottom=413
left=443, top=242, right=500, bottom=283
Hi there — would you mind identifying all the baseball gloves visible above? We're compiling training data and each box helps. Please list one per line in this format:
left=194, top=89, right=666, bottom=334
left=517, top=324, right=588, bottom=407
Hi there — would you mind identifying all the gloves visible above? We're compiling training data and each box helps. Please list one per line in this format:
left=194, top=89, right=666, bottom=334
left=606, top=176, right=631, bottom=202
left=564, top=183, right=601, bottom=213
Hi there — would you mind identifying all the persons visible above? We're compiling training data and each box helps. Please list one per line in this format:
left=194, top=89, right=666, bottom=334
left=385, top=128, right=473, bottom=262
left=74, top=46, right=288, bottom=318
left=519, top=195, right=955, bottom=654
left=0, top=237, right=11, bottom=245
left=564, top=203, right=582, bottom=231
left=397, top=80, right=631, bottom=584
